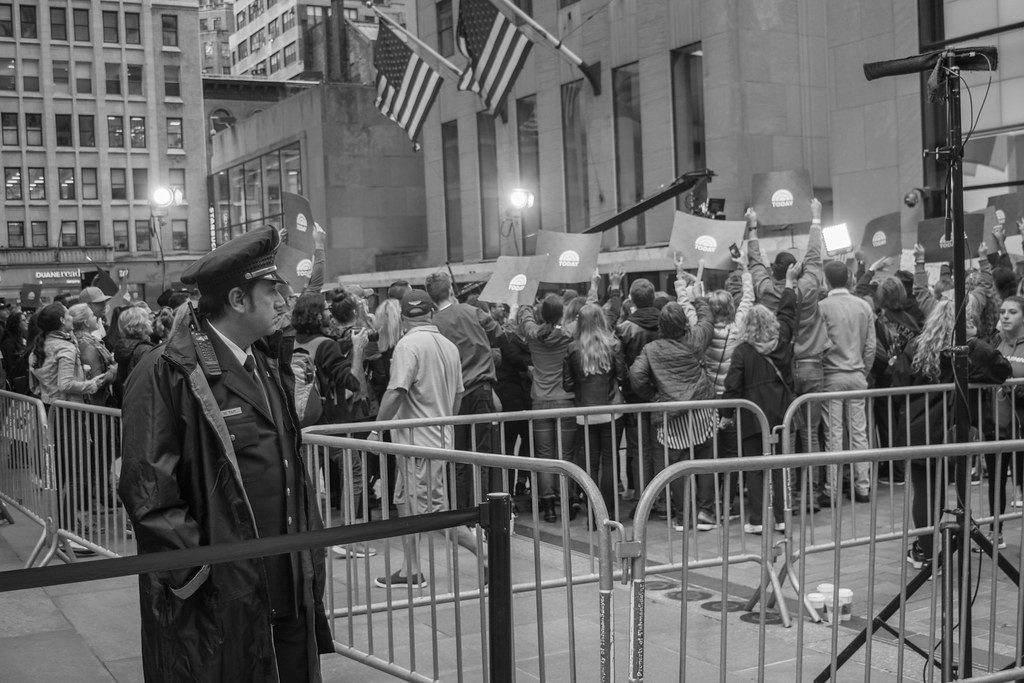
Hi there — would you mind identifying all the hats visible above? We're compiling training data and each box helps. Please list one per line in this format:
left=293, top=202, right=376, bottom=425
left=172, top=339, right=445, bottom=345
left=0, top=299, right=11, bottom=310
left=347, top=284, right=374, bottom=299
left=401, top=290, right=432, bottom=318
left=181, top=224, right=287, bottom=288
left=78, top=286, right=113, bottom=303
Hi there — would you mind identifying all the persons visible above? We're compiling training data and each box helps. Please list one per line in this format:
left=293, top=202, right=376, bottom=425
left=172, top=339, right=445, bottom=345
left=367, top=290, right=489, bottom=590
left=119, top=225, right=335, bottom=683
left=518, top=198, right=1024, bottom=533
left=120, top=222, right=520, bottom=519
left=891, top=289, right=1013, bottom=580
left=971, top=297, right=1023, bottom=552
left=0, top=285, right=119, bottom=556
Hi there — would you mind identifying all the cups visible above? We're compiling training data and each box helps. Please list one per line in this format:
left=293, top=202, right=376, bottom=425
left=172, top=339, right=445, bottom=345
left=839, top=589, right=854, bottom=621
left=808, top=592, right=825, bottom=621
left=816, top=582, right=835, bottom=598
left=827, top=597, right=842, bottom=625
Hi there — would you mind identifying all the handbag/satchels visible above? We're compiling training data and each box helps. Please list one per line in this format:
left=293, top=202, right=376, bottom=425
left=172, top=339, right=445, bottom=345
left=787, top=392, right=806, bottom=432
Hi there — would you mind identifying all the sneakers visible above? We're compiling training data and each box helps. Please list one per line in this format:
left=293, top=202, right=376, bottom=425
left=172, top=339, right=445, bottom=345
left=908, top=540, right=943, bottom=580
left=973, top=534, right=1006, bottom=552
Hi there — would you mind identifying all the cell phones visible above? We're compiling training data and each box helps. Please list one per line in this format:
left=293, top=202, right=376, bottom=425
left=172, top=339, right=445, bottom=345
left=728, top=243, right=741, bottom=259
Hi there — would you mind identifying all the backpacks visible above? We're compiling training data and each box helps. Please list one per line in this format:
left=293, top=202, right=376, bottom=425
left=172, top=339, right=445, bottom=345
left=290, top=338, right=334, bottom=428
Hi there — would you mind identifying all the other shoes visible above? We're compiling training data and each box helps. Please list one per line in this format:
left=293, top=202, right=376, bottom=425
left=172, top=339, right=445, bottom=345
left=482, top=567, right=490, bottom=584
left=333, top=540, right=377, bottom=557
left=375, top=568, right=427, bottom=588
left=512, top=467, right=981, bottom=533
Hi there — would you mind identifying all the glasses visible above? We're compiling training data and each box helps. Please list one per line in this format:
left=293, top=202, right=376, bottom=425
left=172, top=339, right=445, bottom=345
left=325, top=305, right=333, bottom=312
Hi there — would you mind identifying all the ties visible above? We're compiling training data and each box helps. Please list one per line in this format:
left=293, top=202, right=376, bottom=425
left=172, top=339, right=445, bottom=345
left=243, top=353, right=263, bottom=390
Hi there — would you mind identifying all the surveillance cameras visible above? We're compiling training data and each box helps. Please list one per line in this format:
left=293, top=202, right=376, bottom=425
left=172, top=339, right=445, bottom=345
left=904, top=192, right=919, bottom=208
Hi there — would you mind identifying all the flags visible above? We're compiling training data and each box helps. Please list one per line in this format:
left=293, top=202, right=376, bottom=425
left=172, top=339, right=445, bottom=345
left=455, top=1, right=533, bottom=120
left=372, top=18, right=444, bottom=143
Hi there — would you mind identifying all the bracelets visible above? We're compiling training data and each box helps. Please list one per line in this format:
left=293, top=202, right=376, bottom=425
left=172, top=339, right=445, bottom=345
left=371, top=430, right=379, bottom=435
